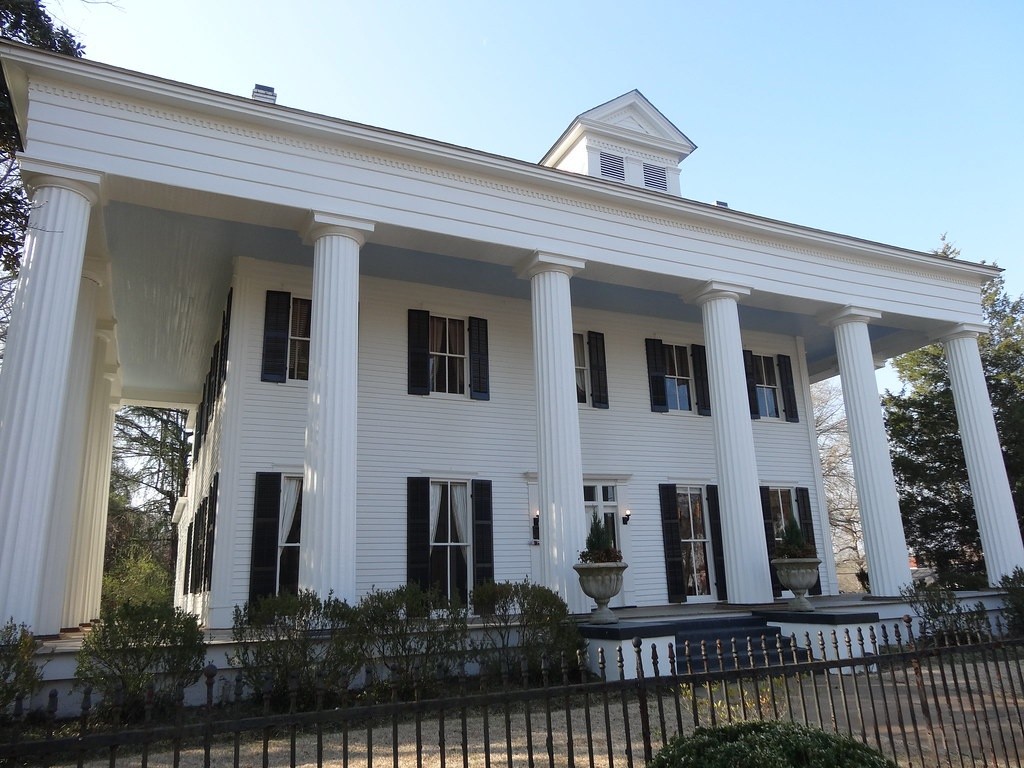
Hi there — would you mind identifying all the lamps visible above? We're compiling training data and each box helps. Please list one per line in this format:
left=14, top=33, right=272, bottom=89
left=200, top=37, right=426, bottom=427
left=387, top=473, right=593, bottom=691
left=623, top=510, right=631, bottom=525
left=534, top=510, right=539, bottom=522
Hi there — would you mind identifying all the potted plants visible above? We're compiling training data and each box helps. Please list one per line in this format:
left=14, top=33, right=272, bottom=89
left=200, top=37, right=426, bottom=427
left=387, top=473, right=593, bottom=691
left=573, top=505, right=629, bottom=625
left=770, top=517, right=823, bottom=612
